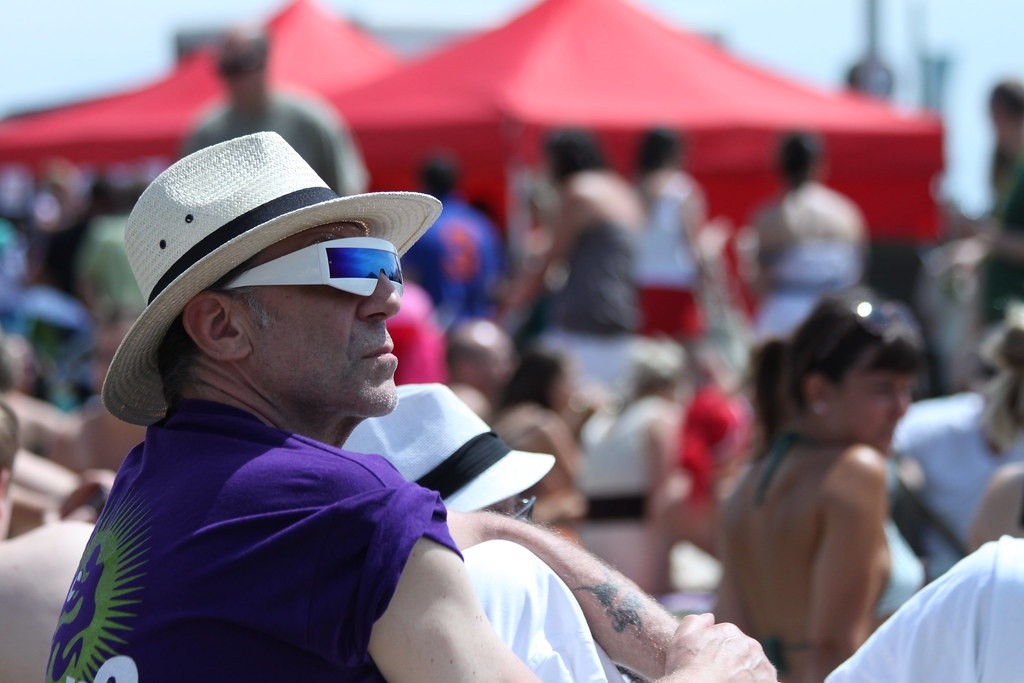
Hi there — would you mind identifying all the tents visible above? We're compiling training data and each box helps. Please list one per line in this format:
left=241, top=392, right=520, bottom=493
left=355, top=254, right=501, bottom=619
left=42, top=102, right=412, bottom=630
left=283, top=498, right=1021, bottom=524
left=323, top=0, right=945, bottom=242
left=0, top=0, right=406, bottom=163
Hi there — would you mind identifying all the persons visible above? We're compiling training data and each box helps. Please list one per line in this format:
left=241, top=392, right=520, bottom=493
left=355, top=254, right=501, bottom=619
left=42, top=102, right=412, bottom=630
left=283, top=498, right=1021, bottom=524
left=1, top=26, right=1024, bottom=683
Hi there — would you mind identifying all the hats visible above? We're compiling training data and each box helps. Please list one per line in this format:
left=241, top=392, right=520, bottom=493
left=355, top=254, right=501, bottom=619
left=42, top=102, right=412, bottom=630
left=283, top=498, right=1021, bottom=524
left=342, top=383, right=556, bottom=511
left=102, top=130, right=444, bottom=427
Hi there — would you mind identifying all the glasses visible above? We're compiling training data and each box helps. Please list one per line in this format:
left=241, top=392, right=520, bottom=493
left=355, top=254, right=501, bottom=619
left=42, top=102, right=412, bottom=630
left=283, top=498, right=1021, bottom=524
left=211, top=236, right=404, bottom=298
